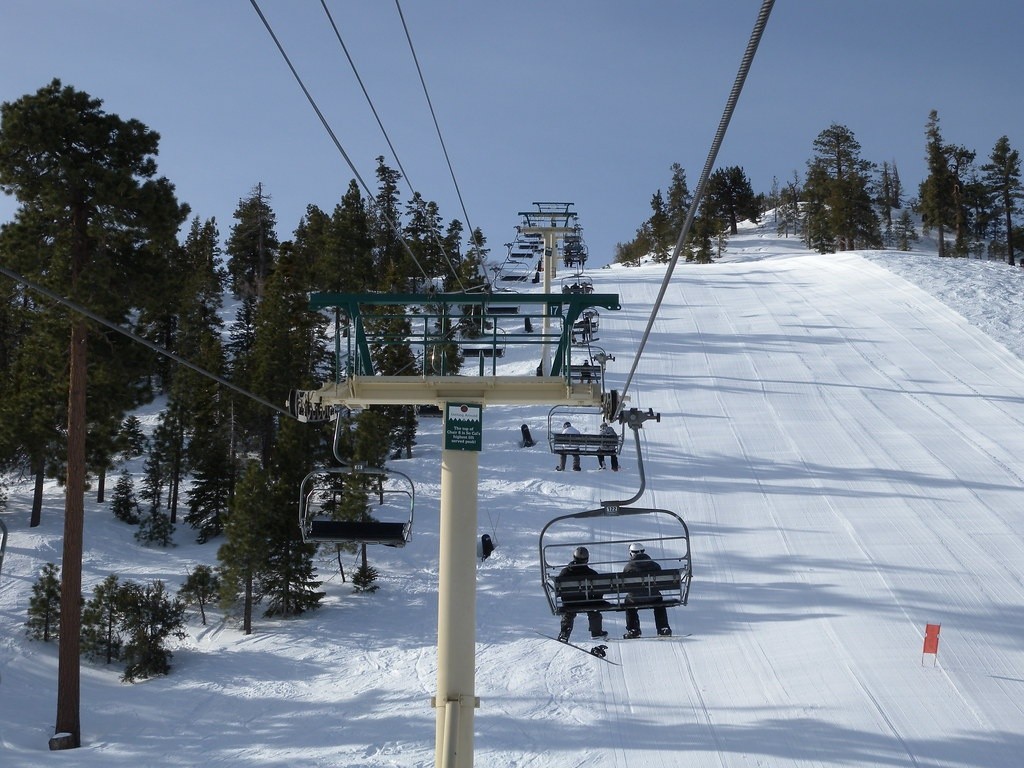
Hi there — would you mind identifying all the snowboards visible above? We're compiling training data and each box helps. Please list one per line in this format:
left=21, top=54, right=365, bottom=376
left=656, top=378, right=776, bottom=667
left=587, top=468, right=628, bottom=472
left=608, top=633, right=693, bottom=641
left=536, top=632, right=620, bottom=667
left=577, top=338, right=599, bottom=345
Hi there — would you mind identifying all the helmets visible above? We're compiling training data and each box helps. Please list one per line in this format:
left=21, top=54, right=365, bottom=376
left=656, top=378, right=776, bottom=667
left=573, top=547, right=589, bottom=562
left=630, top=542, right=645, bottom=556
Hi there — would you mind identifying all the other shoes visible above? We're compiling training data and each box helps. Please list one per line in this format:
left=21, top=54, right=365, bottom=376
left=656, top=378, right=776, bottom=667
left=590, top=630, right=609, bottom=640
left=599, top=467, right=606, bottom=470
left=558, top=633, right=568, bottom=642
left=612, top=468, right=618, bottom=471
left=556, top=466, right=561, bottom=471
left=576, top=468, right=581, bottom=471
left=658, top=629, right=672, bottom=636
left=623, top=630, right=641, bottom=639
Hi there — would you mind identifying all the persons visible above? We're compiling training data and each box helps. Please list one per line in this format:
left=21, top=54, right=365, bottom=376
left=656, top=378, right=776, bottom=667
left=562, top=282, right=580, bottom=294
left=564, top=250, right=574, bottom=267
left=556, top=422, right=582, bottom=471
left=623, top=543, right=672, bottom=639
left=554, top=547, right=608, bottom=643
left=580, top=360, right=592, bottom=384
left=597, top=423, right=619, bottom=472
left=572, top=317, right=591, bottom=344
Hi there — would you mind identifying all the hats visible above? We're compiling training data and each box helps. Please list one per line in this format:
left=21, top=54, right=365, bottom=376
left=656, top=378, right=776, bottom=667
left=564, top=422, right=571, bottom=428
left=600, top=423, right=608, bottom=428
